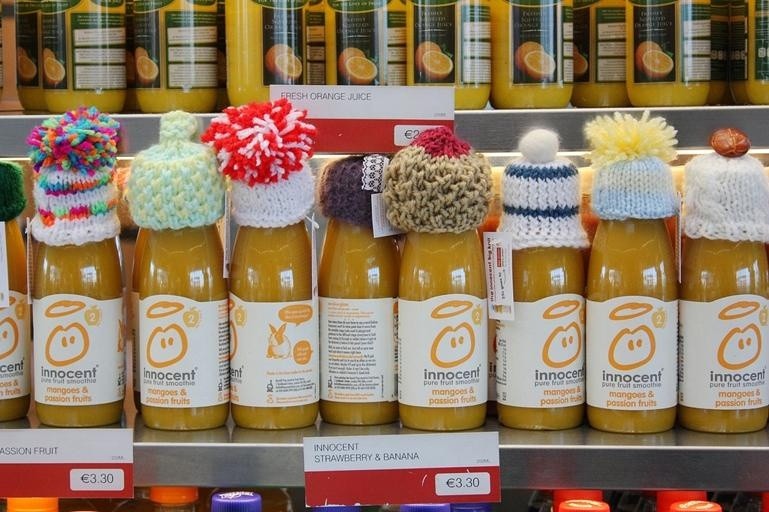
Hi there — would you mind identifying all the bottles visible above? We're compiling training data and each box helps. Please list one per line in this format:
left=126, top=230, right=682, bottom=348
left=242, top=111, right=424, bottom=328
left=33, top=165, right=126, bottom=428
left=586, top=161, right=679, bottom=433
left=398, top=163, right=489, bottom=433
left=230, top=157, right=321, bottom=429
left=0, top=163, right=32, bottom=424
left=132, top=162, right=229, bottom=429
left=495, top=162, right=584, bottom=430
left=320, top=157, right=397, bottom=426
left=681, top=163, right=765, bottom=434
left=0, top=0, right=769, bottom=116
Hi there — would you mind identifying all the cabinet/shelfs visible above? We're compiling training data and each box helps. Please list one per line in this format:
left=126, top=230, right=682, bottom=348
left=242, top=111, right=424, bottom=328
left=0, top=107, right=769, bottom=494
